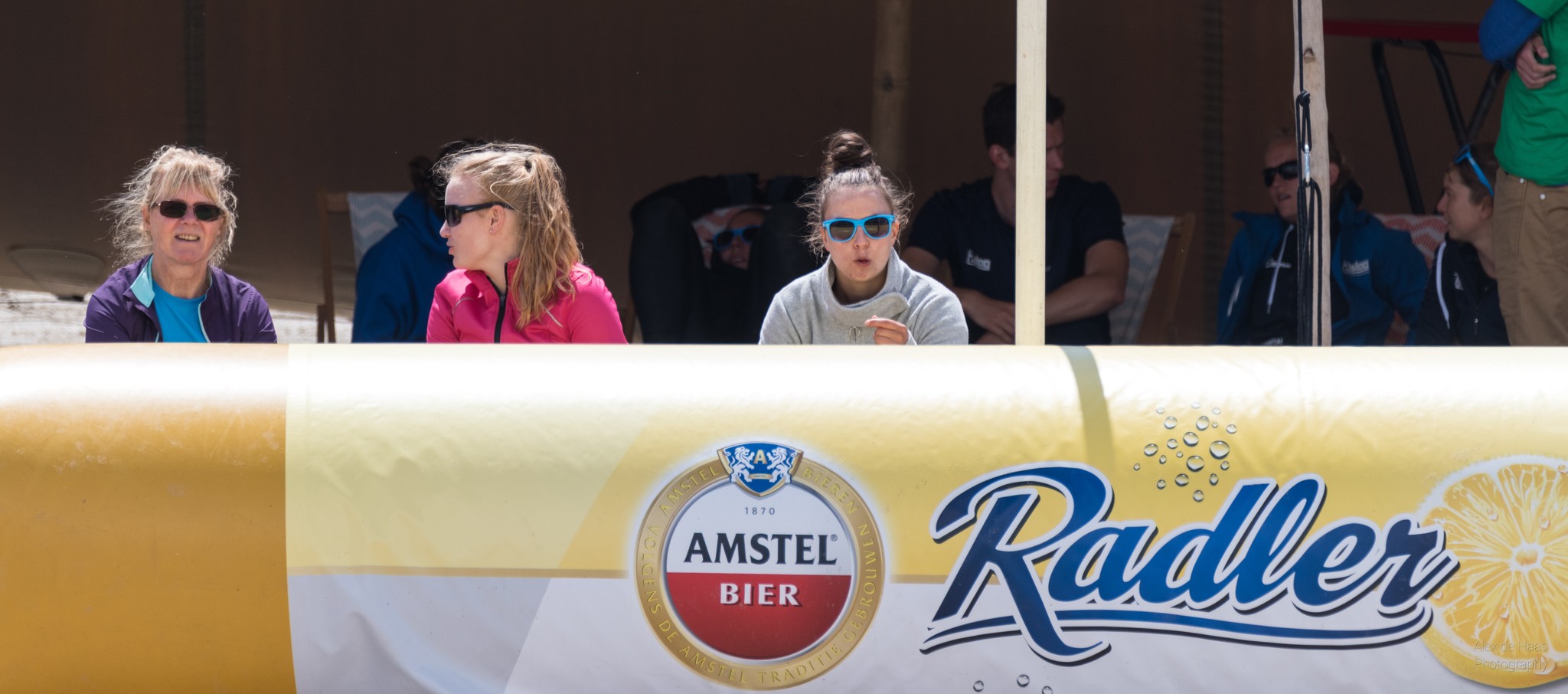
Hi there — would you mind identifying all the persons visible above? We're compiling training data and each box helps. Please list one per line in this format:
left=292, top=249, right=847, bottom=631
left=352, top=137, right=493, bottom=343
left=902, top=82, right=1129, bottom=345
left=83, top=145, right=278, bottom=344
left=427, top=142, right=628, bottom=344
left=1213, top=0, right=1568, bottom=348
left=758, top=130, right=969, bottom=347
left=630, top=173, right=831, bottom=344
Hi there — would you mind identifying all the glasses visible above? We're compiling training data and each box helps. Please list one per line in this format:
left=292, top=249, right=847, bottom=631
left=710, top=223, right=759, bottom=251
left=443, top=200, right=515, bottom=227
left=152, top=198, right=226, bottom=222
left=822, top=213, right=897, bottom=242
left=1453, top=142, right=1495, bottom=198
left=1255, top=161, right=1298, bottom=190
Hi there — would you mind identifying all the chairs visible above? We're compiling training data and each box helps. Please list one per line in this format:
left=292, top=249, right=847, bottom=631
left=1368, top=211, right=1449, bottom=346
left=314, top=184, right=408, bottom=339
left=1110, top=212, right=1197, bottom=345
